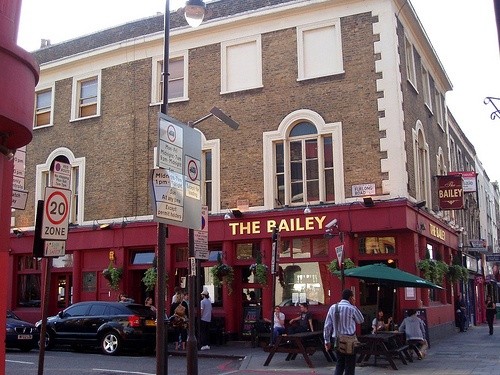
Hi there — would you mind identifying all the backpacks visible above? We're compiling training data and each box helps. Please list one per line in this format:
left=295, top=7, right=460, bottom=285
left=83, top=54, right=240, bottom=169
left=172, top=304, right=188, bottom=329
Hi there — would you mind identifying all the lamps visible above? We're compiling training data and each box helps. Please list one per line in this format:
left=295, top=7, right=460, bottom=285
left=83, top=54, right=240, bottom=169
left=414, top=200, right=426, bottom=207
left=363, top=197, right=374, bottom=206
left=121, top=215, right=129, bottom=228
left=223, top=207, right=232, bottom=219
left=92, top=219, right=100, bottom=230
left=303, top=198, right=311, bottom=214
left=232, top=210, right=242, bottom=217
left=14, top=227, right=25, bottom=238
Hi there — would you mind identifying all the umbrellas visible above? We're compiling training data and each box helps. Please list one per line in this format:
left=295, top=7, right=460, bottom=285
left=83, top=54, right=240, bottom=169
left=334, top=262, right=444, bottom=336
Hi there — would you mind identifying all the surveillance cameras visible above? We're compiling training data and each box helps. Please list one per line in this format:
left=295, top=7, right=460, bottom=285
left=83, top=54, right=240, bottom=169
left=325, top=218, right=337, bottom=228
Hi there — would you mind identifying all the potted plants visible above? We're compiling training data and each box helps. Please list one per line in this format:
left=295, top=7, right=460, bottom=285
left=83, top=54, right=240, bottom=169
left=103, top=265, right=122, bottom=287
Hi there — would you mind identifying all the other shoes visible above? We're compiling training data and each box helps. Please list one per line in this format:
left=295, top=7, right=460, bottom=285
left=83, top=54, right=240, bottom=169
left=201, top=345, right=210, bottom=350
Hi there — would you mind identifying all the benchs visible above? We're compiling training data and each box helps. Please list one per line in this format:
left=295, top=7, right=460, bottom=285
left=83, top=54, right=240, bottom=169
left=394, top=344, right=422, bottom=364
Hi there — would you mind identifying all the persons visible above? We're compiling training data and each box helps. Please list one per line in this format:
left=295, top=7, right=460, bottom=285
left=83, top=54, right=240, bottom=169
left=324, top=289, right=365, bottom=375
left=171, top=287, right=189, bottom=350
left=398, top=309, right=426, bottom=360
left=272, top=305, right=285, bottom=343
left=485, top=295, right=496, bottom=335
left=243, top=291, right=258, bottom=306
left=288, top=303, right=313, bottom=335
left=201, top=290, right=212, bottom=350
left=118, top=293, right=135, bottom=303
left=372, top=310, right=390, bottom=334
left=145, top=297, right=152, bottom=306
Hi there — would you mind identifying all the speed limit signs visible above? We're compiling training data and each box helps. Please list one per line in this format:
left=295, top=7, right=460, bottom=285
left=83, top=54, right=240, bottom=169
left=47, top=190, right=69, bottom=225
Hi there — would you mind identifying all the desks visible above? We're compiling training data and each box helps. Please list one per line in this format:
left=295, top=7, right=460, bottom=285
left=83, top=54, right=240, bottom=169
left=262, top=331, right=336, bottom=368
left=359, top=333, right=401, bottom=370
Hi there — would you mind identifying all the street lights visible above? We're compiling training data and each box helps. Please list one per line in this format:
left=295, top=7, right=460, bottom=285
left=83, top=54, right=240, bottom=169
left=154, top=0, right=207, bottom=373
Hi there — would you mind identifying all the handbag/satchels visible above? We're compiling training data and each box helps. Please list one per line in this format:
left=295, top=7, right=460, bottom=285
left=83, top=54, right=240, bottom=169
left=337, top=335, right=355, bottom=354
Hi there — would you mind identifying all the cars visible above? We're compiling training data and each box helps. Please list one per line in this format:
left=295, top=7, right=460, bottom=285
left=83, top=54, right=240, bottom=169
left=6, top=309, right=40, bottom=353
left=33, top=300, right=184, bottom=356
left=273, top=297, right=335, bottom=325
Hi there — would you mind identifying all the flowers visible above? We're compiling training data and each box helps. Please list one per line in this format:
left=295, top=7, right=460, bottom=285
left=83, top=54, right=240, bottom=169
left=211, top=264, right=233, bottom=287
left=250, top=264, right=269, bottom=286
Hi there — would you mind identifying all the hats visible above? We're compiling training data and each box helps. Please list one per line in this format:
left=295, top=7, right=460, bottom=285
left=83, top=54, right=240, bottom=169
left=200, top=290, right=209, bottom=296
